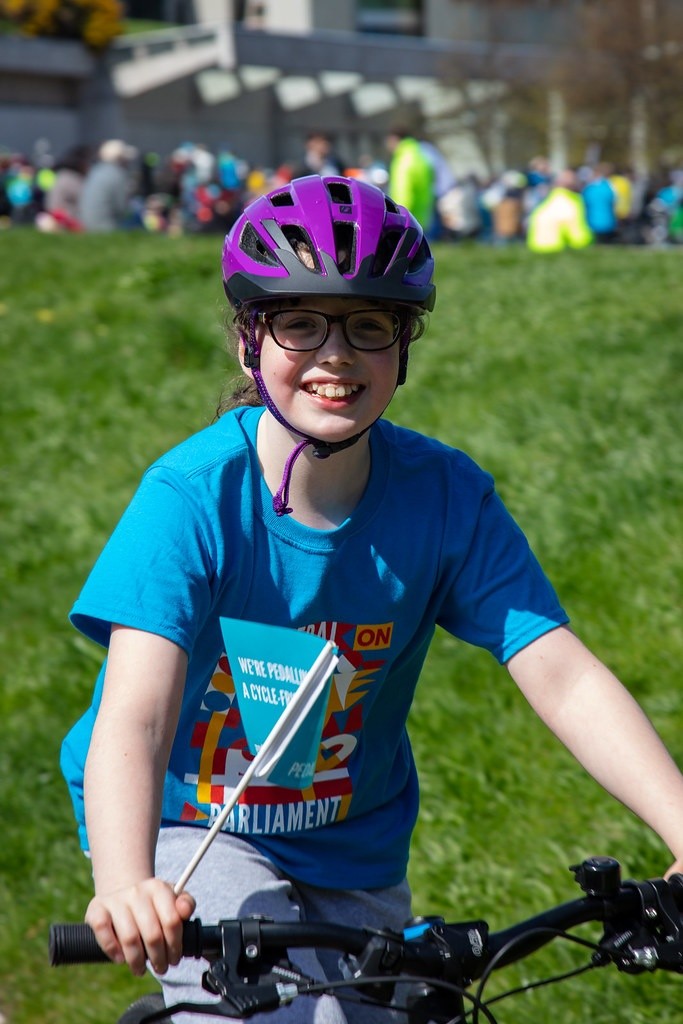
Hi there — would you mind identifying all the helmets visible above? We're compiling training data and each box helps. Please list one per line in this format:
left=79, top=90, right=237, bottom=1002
left=221, top=173, right=437, bottom=313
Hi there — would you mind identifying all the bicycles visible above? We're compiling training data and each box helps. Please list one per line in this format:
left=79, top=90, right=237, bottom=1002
left=45, top=854, right=683, bottom=1024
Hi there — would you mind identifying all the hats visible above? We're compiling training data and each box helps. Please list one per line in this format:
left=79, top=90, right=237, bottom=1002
left=99, top=141, right=140, bottom=163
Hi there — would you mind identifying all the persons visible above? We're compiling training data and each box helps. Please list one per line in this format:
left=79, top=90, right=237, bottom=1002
left=383, top=123, right=438, bottom=238
left=1, top=133, right=683, bottom=252
left=58, top=173, right=683, bottom=1024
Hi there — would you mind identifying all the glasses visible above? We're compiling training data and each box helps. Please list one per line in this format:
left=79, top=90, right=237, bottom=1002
left=251, top=309, right=405, bottom=354
